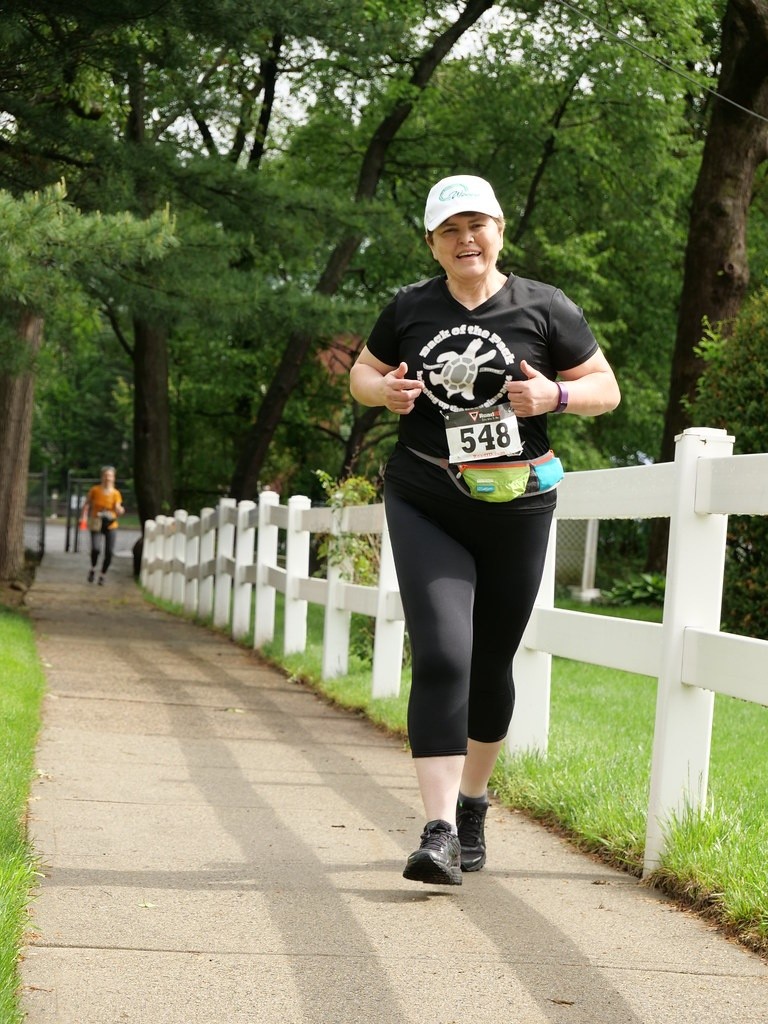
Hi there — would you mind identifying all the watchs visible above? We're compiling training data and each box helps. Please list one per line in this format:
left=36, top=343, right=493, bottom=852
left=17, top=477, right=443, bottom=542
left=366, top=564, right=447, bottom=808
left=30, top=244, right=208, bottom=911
left=549, top=382, right=568, bottom=414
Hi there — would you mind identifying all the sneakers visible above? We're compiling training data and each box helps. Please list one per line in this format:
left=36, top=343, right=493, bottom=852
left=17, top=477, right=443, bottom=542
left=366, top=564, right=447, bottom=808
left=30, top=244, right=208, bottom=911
left=402, top=818, right=464, bottom=887
left=454, top=796, right=492, bottom=872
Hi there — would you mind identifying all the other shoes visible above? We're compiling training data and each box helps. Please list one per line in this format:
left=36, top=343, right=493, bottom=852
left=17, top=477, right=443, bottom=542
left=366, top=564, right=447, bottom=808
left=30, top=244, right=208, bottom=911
left=87, top=569, right=96, bottom=582
left=98, top=576, right=104, bottom=586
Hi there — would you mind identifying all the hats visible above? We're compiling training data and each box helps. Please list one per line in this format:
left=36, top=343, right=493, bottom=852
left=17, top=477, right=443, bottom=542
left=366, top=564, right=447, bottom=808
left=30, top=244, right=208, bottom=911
left=422, top=174, right=504, bottom=230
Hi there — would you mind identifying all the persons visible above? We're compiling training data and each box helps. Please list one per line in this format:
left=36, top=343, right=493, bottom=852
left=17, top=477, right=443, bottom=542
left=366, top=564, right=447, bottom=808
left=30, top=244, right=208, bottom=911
left=80, top=466, right=125, bottom=585
left=350, top=174, right=621, bottom=886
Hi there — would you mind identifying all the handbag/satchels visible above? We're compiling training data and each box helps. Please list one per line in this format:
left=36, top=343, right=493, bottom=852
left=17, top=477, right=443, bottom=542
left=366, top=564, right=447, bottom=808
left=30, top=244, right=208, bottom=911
left=399, top=443, right=565, bottom=500
